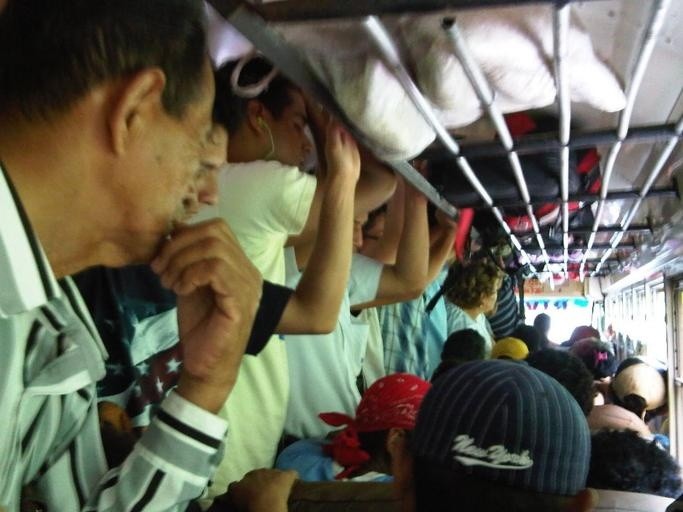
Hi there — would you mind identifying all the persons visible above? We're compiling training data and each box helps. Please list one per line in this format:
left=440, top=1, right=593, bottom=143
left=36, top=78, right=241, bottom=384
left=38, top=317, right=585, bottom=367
left=0, top=2, right=266, bottom=512
left=183, top=57, right=520, bottom=512
left=74, top=72, right=363, bottom=512
left=228, top=312, right=682, bottom=512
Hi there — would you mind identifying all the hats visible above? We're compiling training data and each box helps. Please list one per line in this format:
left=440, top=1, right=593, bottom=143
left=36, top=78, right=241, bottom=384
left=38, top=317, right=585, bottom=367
left=442, top=329, right=485, bottom=362
left=614, top=362, right=666, bottom=422
left=570, top=326, right=600, bottom=344
left=411, top=359, right=592, bottom=498
left=491, top=337, right=530, bottom=362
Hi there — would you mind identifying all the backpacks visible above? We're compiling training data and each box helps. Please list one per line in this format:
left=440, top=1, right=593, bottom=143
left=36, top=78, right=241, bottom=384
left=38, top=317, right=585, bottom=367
left=426, top=109, right=602, bottom=265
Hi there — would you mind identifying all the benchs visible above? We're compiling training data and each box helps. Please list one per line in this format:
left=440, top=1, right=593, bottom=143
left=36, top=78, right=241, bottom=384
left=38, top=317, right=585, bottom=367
left=587, top=404, right=651, bottom=438
left=206, top=480, right=677, bottom=511
